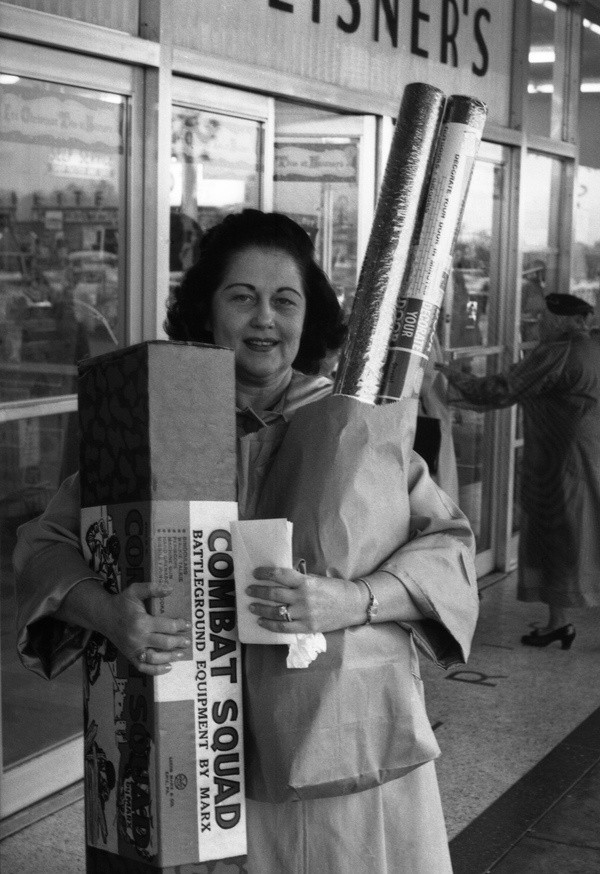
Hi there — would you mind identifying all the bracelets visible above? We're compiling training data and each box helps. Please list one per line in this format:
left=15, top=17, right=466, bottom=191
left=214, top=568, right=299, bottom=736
left=356, top=577, right=379, bottom=626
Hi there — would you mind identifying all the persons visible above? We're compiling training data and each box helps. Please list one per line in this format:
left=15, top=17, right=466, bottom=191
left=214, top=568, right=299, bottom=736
left=431, top=292, right=600, bottom=652
left=11, top=207, right=479, bottom=874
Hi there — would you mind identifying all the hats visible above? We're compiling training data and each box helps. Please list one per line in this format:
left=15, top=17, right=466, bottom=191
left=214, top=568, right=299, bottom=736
left=544, top=292, right=595, bottom=316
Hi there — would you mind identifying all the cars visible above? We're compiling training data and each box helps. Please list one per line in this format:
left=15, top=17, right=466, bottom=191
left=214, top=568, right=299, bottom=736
left=458, top=263, right=600, bottom=345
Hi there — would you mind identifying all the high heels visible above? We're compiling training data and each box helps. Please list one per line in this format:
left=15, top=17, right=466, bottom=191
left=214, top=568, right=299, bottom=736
left=520, top=625, right=576, bottom=650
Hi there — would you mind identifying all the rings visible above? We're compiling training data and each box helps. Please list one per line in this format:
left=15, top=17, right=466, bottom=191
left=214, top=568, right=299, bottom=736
left=278, top=606, right=291, bottom=623
left=138, top=650, right=147, bottom=664
